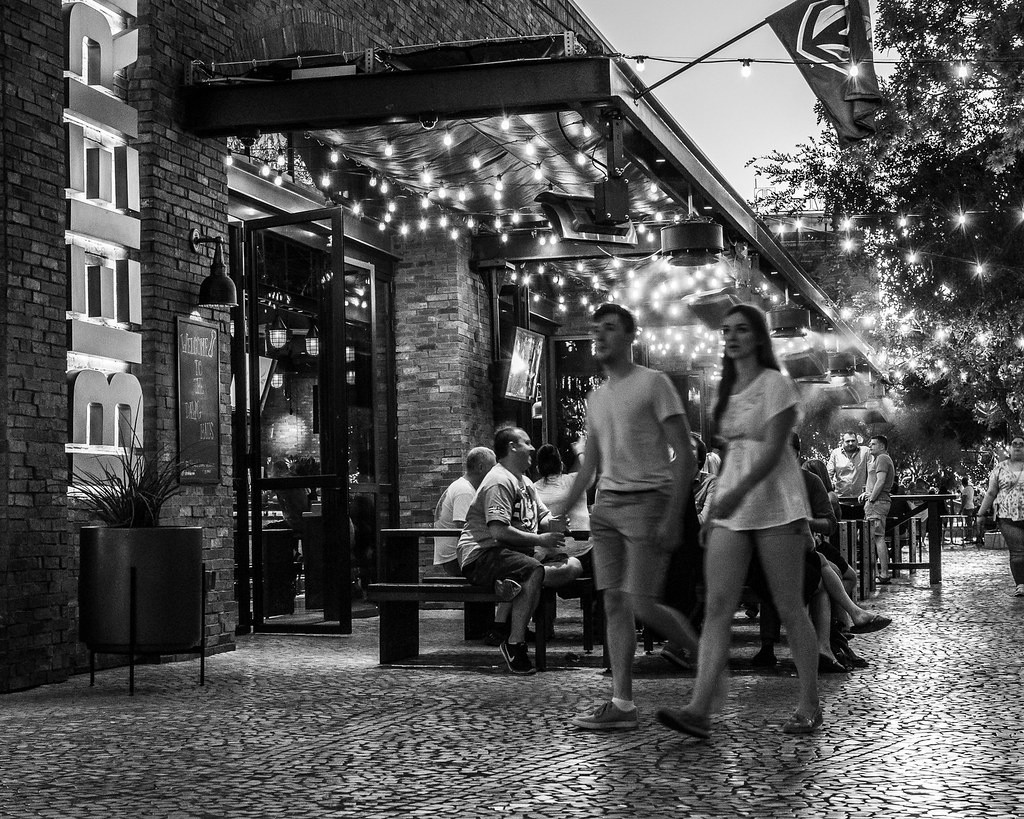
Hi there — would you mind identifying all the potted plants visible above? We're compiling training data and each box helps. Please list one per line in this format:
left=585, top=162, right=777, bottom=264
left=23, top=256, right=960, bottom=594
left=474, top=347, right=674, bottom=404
left=54, top=425, right=210, bottom=655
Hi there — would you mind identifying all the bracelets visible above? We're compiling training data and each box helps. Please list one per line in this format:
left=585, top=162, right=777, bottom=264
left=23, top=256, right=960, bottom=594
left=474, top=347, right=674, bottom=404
left=577, top=452, right=585, bottom=457
left=976, top=514, right=983, bottom=517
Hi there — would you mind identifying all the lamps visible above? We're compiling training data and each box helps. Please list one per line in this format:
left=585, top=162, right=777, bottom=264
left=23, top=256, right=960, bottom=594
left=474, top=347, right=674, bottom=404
left=346, top=369, right=356, bottom=385
left=270, top=372, right=284, bottom=389
left=186, top=228, right=239, bottom=311
left=305, top=322, right=321, bottom=358
left=345, top=342, right=357, bottom=362
left=268, top=311, right=287, bottom=349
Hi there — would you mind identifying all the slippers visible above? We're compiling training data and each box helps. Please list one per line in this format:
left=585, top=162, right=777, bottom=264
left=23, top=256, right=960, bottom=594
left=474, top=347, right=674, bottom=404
left=850, top=615, right=890, bottom=633
left=819, top=655, right=847, bottom=672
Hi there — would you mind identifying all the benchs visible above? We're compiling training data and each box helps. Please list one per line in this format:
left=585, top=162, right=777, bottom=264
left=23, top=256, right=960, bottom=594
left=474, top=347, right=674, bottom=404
left=364, top=566, right=604, bottom=673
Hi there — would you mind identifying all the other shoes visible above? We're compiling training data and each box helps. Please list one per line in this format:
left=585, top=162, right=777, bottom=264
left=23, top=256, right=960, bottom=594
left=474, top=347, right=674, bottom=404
left=835, top=635, right=854, bottom=641
left=746, top=606, right=759, bottom=619
left=744, top=650, right=777, bottom=666
left=831, top=638, right=865, bottom=667
left=485, top=623, right=510, bottom=646
left=524, top=625, right=537, bottom=641
left=782, top=702, right=823, bottom=732
left=655, top=708, right=711, bottom=739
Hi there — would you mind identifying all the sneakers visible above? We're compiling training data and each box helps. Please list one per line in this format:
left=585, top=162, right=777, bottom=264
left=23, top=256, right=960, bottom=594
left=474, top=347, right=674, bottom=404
left=499, top=634, right=536, bottom=674
left=661, top=643, right=690, bottom=668
left=572, top=701, right=637, bottom=729
left=1015, top=583, right=1024, bottom=596
left=495, top=577, right=522, bottom=602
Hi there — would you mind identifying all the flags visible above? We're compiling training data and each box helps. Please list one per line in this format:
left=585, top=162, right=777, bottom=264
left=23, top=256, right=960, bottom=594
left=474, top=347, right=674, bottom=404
left=766, top=0, right=883, bottom=148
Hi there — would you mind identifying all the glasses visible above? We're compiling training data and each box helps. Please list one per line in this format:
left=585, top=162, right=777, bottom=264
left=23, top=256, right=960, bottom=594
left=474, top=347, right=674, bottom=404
left=1011, top=442, right=1023, bottom=447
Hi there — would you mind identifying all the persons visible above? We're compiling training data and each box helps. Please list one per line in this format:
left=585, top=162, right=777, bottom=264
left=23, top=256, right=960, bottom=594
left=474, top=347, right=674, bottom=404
left=655, top=305, right=823, bottom=741
left=889, top=475, right=956, bottom=540
left=558, top=303, right=729, bottom=728
left=958, top=478, right=975, bottom=541
left=456, top=426, right=585, bottom=675
left=690, top=431, right=722, bottom=525
left=263, top=461, right=312, bottom=562
left=973, top=435, right=1024, bottom=596
left=433, top=447, right=496, bottom=576
left=747, top=431, right=895, bottom=672
left=534, top=441, right=596, bottom=542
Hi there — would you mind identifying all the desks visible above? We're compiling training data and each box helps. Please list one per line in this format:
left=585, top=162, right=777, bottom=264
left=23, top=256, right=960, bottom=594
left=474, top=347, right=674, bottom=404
left=820, top=492, right=958, bottom=586
left=379, top=524, right=603, bottom=667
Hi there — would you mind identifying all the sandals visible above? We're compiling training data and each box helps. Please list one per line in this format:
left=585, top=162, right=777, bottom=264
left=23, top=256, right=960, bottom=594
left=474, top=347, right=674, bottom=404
left=875, top=575, right=892, bottom=585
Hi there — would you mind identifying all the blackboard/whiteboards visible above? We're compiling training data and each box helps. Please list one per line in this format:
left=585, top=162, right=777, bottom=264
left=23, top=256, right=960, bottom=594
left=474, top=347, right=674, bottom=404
left=176, top=316, right=223, bottom=485
